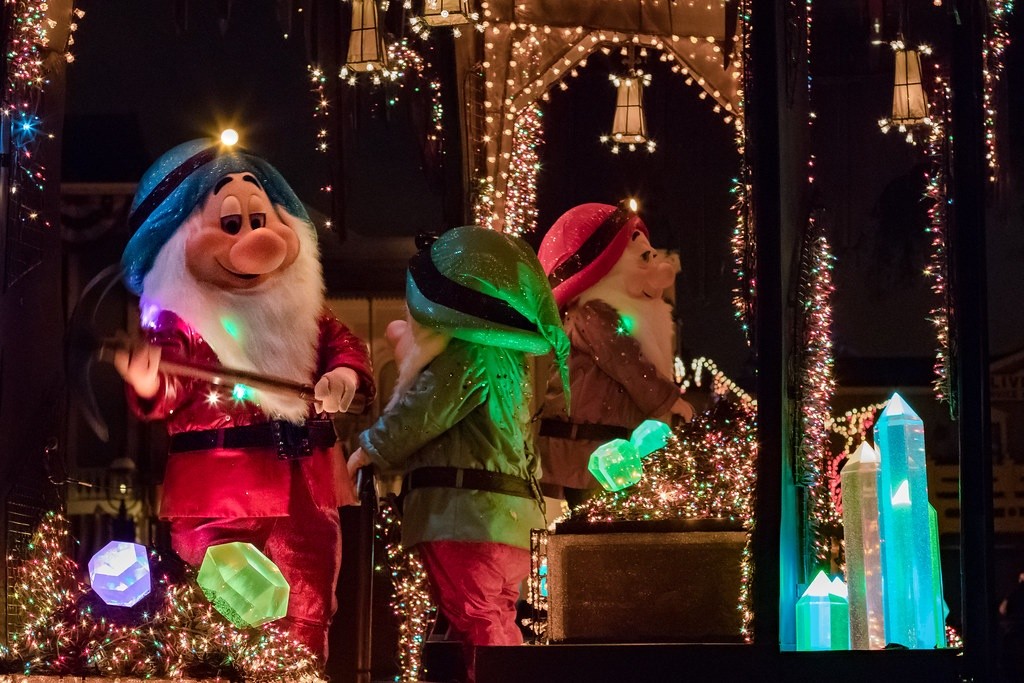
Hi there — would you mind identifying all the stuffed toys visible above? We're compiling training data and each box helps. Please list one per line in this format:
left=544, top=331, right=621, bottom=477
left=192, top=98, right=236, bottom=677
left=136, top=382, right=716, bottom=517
left=536, top=203, right=696, bottom=528
left=113, top=138, right=377, bottom=675
left=346, top=226, right=570, bottom=683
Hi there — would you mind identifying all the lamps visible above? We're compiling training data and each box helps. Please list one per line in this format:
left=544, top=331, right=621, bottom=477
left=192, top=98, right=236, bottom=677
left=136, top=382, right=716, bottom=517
left=877, top=0, right=933, bottom=143
left=598, top=45, right=658, bottom=155
left=338, top=0, right=390, bottom=86
left=409, top=0, right=486, bottom=40
